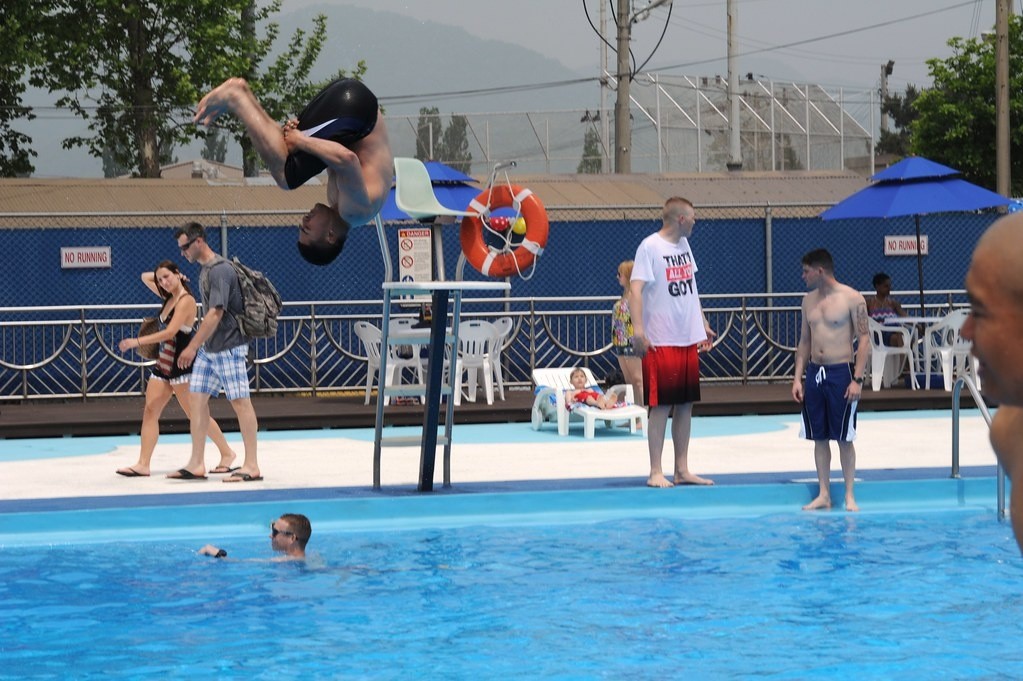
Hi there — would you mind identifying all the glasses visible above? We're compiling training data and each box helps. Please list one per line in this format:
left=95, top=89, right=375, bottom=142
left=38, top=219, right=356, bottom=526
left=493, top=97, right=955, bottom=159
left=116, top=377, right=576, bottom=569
left=272, top=523, right=292, bottom=537
left=178, top=236, right=197, bottom=251
left=617, top=271, right=621, bottom=278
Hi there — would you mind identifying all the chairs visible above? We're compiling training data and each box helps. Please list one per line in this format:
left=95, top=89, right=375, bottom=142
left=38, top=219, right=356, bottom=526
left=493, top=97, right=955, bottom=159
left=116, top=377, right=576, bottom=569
left=855, top=308, right=981, bottom=391
left=531, top=367, right=648, bottom=437
left=354, top=313, right=514, bottom=406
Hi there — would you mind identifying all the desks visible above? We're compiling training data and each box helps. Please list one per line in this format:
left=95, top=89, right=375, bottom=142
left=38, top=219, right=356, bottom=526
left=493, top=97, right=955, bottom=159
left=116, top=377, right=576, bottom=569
left=385, top=326, right=467, bottom=406
left=885, top=315, right=949, bottom=390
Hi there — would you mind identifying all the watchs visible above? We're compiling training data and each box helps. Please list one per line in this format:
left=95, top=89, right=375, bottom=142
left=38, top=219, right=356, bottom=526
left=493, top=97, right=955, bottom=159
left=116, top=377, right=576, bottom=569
left=852, top=376, right=864, bottom=383
left=215, top=549, right=227, bottom=558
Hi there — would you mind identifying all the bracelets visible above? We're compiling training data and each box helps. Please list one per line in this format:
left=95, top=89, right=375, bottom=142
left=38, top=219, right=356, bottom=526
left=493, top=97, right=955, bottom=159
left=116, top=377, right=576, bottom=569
left=137, top=337, right=141, bottom=346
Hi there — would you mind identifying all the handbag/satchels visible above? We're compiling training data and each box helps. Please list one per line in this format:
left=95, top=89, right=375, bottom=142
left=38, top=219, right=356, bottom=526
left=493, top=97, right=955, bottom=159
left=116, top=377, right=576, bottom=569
left=136, top=317, right=160, bottom=360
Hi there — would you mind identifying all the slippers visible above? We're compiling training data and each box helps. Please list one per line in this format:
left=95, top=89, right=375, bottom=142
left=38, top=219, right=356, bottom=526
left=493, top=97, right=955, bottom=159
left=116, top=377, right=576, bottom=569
left=116, top=467, right=150, bottom=476
left=222, top=472, right=263, bottom=483
left=208, top=466, right=241, bottom=473
left=165, top=469, right=208, bottom=479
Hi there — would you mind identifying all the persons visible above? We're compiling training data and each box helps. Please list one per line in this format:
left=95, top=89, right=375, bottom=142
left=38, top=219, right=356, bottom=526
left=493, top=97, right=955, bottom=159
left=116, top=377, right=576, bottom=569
left=865, top=273, right=922, bottom=379
left=791, top=249, right=870, bottom=512
left=627, top=197, right=717, bottom=487
left=565, top=368, right=617, bottom=411
left=198, top=514, right=312, bottom=563
left=194, top=76, right=393, bottom=266
left=116, top=221, right=264, bottom=482
left=959, top=210, right=1023, bottom=555
left=612, top=260, right=649, bottom=429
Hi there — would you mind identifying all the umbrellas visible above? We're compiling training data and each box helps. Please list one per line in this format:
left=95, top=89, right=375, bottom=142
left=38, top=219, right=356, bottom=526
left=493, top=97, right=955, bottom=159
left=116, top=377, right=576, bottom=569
left=817, top=155, right=1018, bottom=336
left=380, top=156, right=523, bottom=281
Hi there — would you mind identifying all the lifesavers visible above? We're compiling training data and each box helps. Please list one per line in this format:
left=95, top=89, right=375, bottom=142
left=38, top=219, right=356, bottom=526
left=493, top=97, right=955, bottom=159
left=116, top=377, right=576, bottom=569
left=458, top=185, right=550, bottom=278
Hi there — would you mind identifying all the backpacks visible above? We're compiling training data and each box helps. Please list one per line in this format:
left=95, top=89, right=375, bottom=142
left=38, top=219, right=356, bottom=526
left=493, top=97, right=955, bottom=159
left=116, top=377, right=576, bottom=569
left=202, top=256, right=282, bottom=337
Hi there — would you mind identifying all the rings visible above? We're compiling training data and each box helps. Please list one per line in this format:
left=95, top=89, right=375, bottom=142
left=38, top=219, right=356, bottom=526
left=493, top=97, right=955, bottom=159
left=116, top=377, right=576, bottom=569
left=289, top=122, right=294, bottom=128
left=123, top=343, right=126, bottom=347
